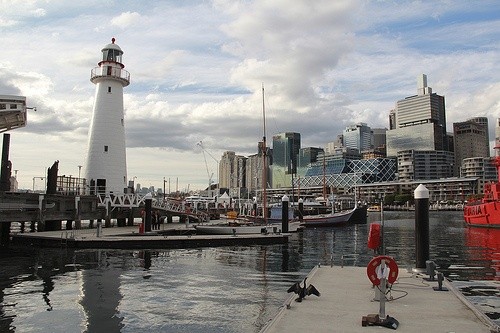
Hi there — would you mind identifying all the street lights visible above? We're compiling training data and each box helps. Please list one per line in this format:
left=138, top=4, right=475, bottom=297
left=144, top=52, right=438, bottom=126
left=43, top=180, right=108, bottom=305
left=78, top=165, right=82, bottom=196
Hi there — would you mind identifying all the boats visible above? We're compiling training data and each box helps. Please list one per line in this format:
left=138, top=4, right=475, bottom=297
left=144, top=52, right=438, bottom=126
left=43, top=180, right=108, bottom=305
left=368, top=207, right=380, bottom=212
left=192, top=222, right=305, bottom=234
left=241, top=193, right=367, bottom=226
left=463, top=142, right=499, bottom=229
left=184, top=191, right=243, bottom=211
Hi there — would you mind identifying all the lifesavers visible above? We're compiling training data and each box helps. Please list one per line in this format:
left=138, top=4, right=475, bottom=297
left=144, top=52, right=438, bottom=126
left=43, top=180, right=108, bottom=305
left=367, top=256, right=399, bottom=285
